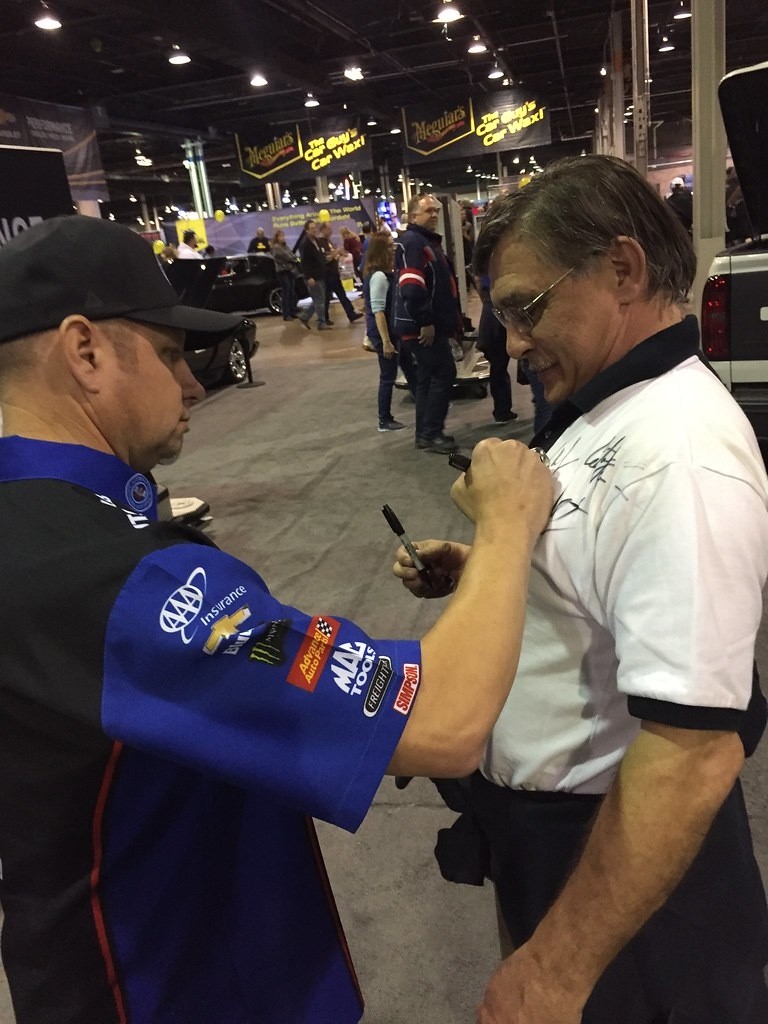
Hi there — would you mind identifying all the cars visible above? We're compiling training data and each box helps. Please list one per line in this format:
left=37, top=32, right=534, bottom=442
left=182, top=319, right=260, bottom=388
left=160, top=252, right=311, bottom=315
left=699, top=59, right=768, bottom=414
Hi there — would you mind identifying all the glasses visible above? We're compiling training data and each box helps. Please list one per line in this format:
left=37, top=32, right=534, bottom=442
left=492, top=246, right=606, bottom=338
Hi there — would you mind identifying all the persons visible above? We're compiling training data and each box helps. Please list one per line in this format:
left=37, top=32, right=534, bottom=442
left=198, top=246, right=215, bottom=257
left=666, top=177, right=693, bottom=230
left=248, top=227, right=271, bottom=252
left=360, top=192, right=550, bottom=455
left=297, top=221, right=363, bottom=330
left=273, top=230, right=299, bottom=321
left=394, top=157, right=768, bottom=1024
left=0, top=217, right=553, bottom=1024
left=724, top=165, right=753, bottom=243
left=176, top=229, right=204, bottom=259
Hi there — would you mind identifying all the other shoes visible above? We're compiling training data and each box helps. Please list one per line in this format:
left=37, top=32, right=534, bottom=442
left=290, top=315, right=299, bottom=318
left=300, top=318, right=311, bottom=330
left=318, top=325, right=332, bottom=330
left=495, top=410, right=518, bottom=422
left=326, top=320, right=333, bottom=325
left=350, top=313, right=363, bottom=322
left=378, top=421, right=408, bottom=432
left=284, top=316, right=293, bottom=321
left=414, top=431, right=459, bottom=453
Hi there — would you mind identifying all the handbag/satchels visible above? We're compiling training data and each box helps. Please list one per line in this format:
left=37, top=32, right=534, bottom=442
left=362, top=328, right=377, bottom=352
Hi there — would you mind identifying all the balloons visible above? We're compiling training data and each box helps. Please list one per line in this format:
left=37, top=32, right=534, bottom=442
left=319, top=210, right=330, bottom=222
left=153, top=240, right=164, bottom=254
left=519, top=178, right=530, bottom=188
left=215, top=210, right=225, bottom=222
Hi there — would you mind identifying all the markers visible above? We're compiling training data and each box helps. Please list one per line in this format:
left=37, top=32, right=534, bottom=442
left=449, top=453, right=473, bottom=472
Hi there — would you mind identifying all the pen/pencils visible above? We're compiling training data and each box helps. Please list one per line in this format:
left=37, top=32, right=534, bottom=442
left=382, top=503, right=434, bottom=592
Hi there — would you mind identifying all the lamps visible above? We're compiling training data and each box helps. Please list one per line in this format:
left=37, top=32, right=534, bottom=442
left=390, top=124, right=401, bottom=134
left=467, top=35, right=487, bottom=53
left=168, top=43, right=191, bottom=64
left=135, top=149, right=152, bottom=167
left=304, top=90, right=320, bottom=108
left=366, top=115, right=377, bottom=126
left=34, top=0, right=63, bottom=29
left=673, top=0, right=693, bottom=20
left=343, top=60, right=364, bottom=80
left=658, top=35, right=675, bottom=52
left=487, top=61, right=504, bottom=79
left=438, top=0, right=460, bottom=20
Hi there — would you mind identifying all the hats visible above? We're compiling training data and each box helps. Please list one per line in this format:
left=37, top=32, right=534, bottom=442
left=1, top=216, right=246, bottom=351
